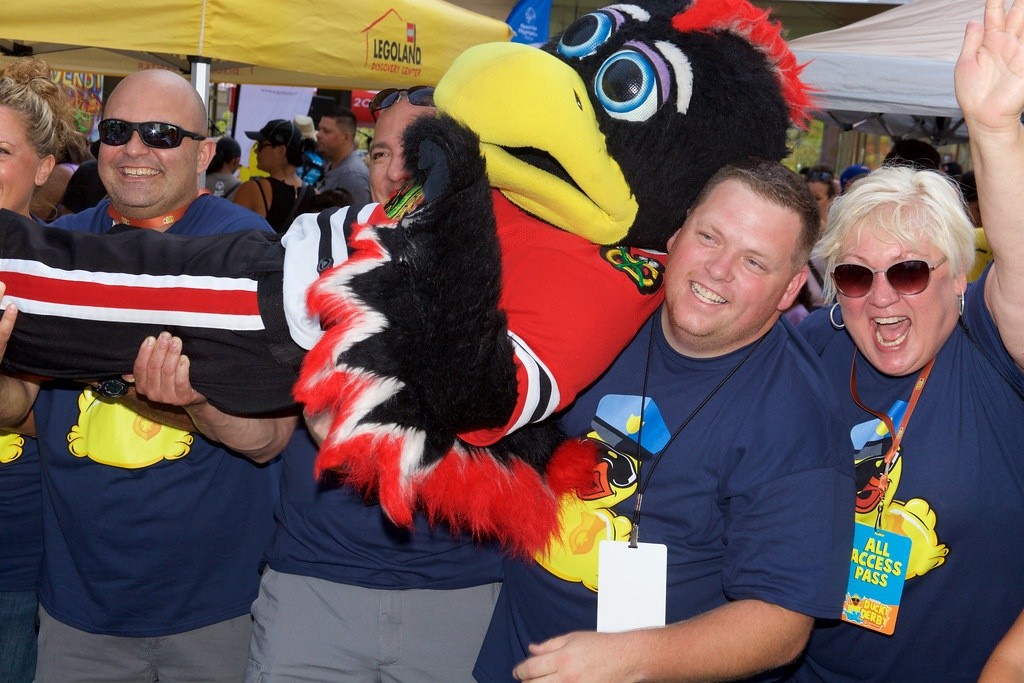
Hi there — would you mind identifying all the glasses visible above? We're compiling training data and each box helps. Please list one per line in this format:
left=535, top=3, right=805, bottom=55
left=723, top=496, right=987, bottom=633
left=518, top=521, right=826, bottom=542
left=830, top=256, right=948, bottom=298
left=369, top=86, right=436, bottom=123
left=804, top=171, right=832, bottom=183
left=98, top=118, right=206, bottom=149
left=257, top=140, right=272, bottom=151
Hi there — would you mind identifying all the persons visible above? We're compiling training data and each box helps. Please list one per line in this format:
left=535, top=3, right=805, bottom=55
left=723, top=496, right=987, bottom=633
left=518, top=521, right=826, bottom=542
left=0, top=79, right=61, bottom=683
left=198, top=103, right=375, bottom=239
left=246, top=88, right=514, bottom=683
left=0, top=67, right=296, bottom=683
left=779, top=137, right=993, bottom=333
left=791, top=0, right=1022, bottom=683
left=472, top=156, right=855, bottom=682
left=32, top=130, right=108, bottom=231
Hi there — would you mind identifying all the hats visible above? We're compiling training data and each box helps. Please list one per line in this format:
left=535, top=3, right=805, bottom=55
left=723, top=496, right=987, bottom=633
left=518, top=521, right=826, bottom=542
left=244, top=119, right=302, bottom=148
left=840, top=164, right=870, bottom=194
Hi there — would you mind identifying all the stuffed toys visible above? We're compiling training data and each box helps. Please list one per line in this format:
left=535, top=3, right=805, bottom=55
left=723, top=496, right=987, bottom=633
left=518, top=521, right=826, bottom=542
left=0, top=1, right=809, bottom=566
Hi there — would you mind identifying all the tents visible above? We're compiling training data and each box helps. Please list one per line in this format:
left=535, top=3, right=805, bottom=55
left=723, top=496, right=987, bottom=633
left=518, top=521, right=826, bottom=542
left=0, top=2, right=514, bottom=132
left=781, top=0, right=1022, bottom=142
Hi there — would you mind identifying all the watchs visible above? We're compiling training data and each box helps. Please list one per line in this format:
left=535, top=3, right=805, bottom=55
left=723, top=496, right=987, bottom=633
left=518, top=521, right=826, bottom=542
left=90, top=376, right=129, bottom=399
